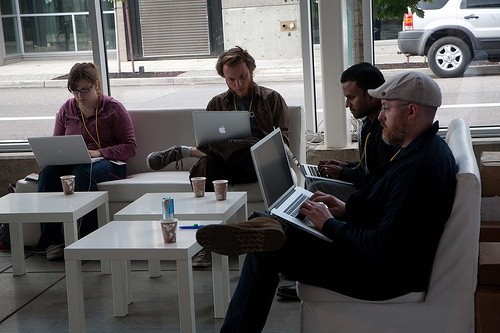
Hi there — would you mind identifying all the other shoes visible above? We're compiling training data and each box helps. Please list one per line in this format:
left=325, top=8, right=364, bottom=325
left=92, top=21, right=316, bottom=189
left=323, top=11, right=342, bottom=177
left=277, top=284, right=301, bottom=301
left=192, top=248, right=212, bottom=268
left=195, top=216, right=286, bottom=256
left=45, top=244, right=63, bottom=262
left=146, top=145, right=183, bottom=171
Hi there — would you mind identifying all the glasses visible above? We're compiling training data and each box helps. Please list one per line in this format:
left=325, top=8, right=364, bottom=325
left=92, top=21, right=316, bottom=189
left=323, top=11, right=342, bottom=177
left=72, top=82, right=94, bottom=95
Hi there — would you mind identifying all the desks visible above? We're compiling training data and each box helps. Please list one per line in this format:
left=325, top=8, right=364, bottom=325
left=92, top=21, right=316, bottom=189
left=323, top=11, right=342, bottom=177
left=63, top=220, right=230, bottom=333
left=0, top=190, right=111, bottom=277
left=113, top=191, right=248, bottom=319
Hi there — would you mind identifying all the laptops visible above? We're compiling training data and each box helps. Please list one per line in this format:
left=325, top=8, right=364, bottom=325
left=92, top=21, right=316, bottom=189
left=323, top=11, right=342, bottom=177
left=192, top=110, right=253, bottom=147
left=273, top=126, right=354, bottom=185
left=250, top=127, right=348, bottom=242
left=28, top=135, right=103, bottom=166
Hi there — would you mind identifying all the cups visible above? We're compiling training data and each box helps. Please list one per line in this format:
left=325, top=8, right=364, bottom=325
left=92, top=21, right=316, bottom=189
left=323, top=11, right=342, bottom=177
left=60, top=175, right=76, bottom=194
left=160, top=218, right=178, bottom=244
left=212, top=180, right=228, bottom=200
left=191, top=177, right=206, bottom=197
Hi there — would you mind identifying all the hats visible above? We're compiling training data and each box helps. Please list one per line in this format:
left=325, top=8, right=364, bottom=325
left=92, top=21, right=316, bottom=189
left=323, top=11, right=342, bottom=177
left=367, top=71, right=442, bottom=107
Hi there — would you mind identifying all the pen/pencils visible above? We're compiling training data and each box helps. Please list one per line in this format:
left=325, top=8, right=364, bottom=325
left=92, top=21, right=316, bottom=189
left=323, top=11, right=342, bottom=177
left=179, top=223, right=204, bottom=230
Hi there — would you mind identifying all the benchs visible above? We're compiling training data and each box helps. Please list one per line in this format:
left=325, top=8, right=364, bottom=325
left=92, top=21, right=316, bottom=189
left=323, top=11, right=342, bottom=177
left=17, top=107, right=305, bottom=247
left=295, top=119, right=481, bottom=333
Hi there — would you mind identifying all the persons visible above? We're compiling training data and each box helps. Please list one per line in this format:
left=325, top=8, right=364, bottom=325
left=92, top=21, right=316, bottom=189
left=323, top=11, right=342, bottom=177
left=276, top=61, right=385, bottom=302
left=146, top=46, right=290, bottom=267
left=37, top=62, right=137, bottom=265
left=196, top=71, right=458, bottom=333
